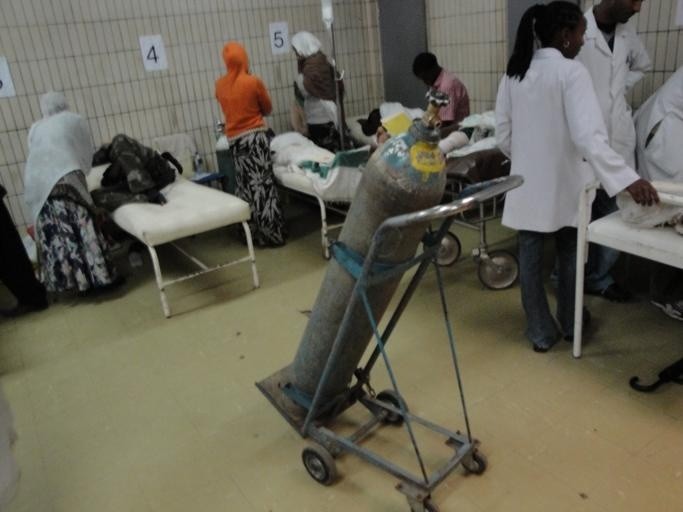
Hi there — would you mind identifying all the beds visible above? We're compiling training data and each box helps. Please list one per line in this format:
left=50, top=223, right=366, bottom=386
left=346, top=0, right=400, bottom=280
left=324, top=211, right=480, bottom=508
left=425, top=139, right=524, bottom=289
left=573, top=161, right=683, bottom=368
left=262, top=134, right=363, bottom=259
left=348, top=105, right=502, bottom=184
left=80, top=146, right=259, bottom=316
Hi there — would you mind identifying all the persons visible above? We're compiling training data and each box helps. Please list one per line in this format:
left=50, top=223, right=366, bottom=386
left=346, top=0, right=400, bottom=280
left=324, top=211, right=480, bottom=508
left=551, top=0, right=649, bottom=302
left=633, top=60, right=683, bottom=181
left=290, top=30, right=344, bottom=152
left=1, top=183, right=48, bottom=309
left=23, top=91, right=127, bottom=301
left=89, top=133, right=174, bottom=205
left=359, top=109, right=390, bottom=144
left=495, top=0, right=659, bottom=353
left=413, top=52, right=470, bottom=140
left=214, top=41, right=285, bottom=247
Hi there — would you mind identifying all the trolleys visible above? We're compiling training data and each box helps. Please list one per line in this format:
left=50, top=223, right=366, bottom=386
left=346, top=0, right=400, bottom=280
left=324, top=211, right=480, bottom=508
left=252, top=170, right=525, bottom=512
left=420, top=161, right=521, bottom=292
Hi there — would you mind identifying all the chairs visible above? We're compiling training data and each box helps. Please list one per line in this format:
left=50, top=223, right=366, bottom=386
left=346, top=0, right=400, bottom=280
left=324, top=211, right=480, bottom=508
left=155, top=132, right=221, bottom=191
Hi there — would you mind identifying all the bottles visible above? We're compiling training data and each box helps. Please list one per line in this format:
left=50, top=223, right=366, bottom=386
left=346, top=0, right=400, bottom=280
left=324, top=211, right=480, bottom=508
left=194, top=152, right=202, bottom=175
